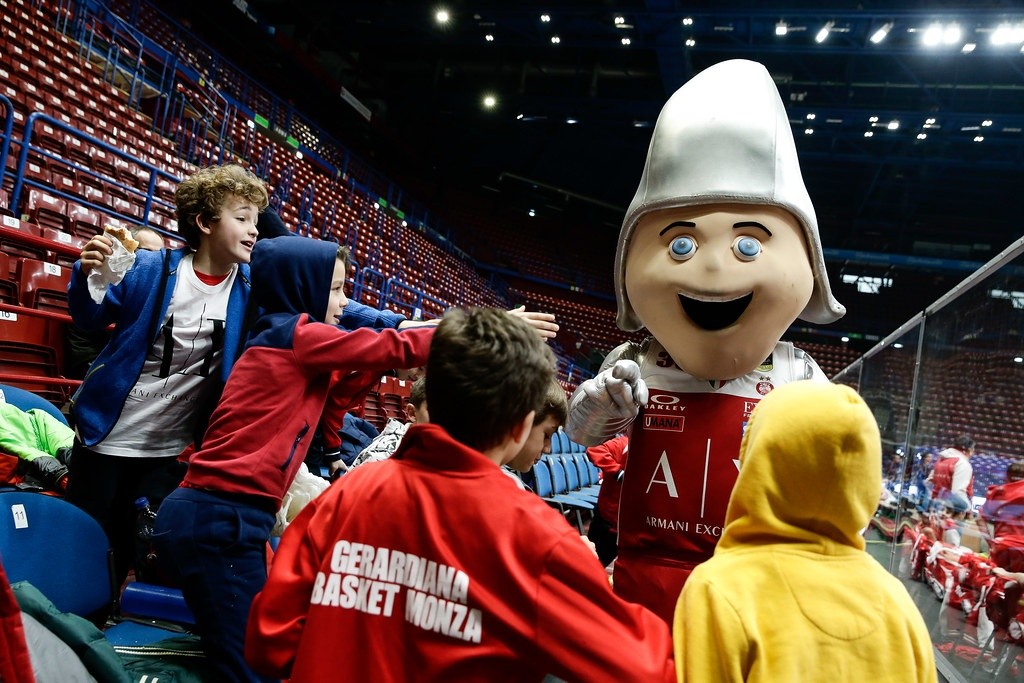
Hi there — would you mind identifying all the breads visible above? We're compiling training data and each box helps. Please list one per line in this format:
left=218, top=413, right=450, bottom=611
left=105, top=224, right=140, bottom=252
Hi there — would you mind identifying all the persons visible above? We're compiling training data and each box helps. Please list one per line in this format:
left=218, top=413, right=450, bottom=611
left=154, top=236, right=560, bottom=683
left=245, top=308, right=676, bottom=683
left=67, top=164, right=443, bottom=632
left=924, top=436, right=1024, bottom=662
left=881, top=450, right=934, bottom=490
left=673, top=382, right=938, bottom=683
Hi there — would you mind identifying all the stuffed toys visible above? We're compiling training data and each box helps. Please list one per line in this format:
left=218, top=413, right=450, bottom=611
left=561, top=58, right=846, bottom=636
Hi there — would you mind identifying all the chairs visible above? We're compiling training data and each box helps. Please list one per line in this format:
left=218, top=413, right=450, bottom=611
left=0, top=0, right=1024, bottom=683
